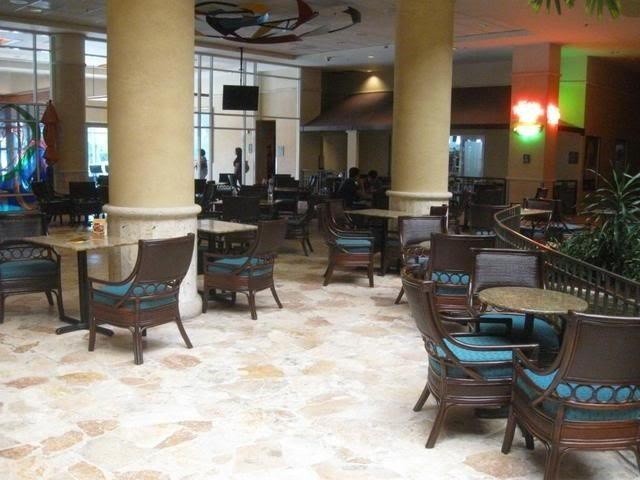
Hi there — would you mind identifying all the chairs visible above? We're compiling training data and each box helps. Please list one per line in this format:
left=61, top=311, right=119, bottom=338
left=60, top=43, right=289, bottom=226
left=194, top=169, right=393, bottom=322
left=430, top=204, right=449, bottom=218
left=502, top=309, right=639, bottom=479
left=399, top=263, right=540, bottom=450
left=395, top=214, right=450, bottom=304
left=450, top=173, right=579, bottom=232
left=86, top=232, right=196, bottom=366
left=467, top=247, right=565, bottom=356
left=1, top=212, right=63, bottom=326
left=425, top=230, right=498, bottom=295
left=30, top=174, right=108, bottom=228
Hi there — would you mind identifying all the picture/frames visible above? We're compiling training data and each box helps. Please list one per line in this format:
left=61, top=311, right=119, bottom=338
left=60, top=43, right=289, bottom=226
left=611, top=136, right=629, bottom=175
left=581, top=134, right=602, bottom=195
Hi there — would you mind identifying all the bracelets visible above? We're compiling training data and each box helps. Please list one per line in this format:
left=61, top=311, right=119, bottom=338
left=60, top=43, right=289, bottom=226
left=375, top=189, right=377, bottom=192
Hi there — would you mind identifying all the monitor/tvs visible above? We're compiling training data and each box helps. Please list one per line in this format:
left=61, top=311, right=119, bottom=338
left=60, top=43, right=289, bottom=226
left=223, top=85, right=259, bottom=110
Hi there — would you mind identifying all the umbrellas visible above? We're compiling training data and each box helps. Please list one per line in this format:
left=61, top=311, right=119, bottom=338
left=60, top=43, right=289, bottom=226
left=41, top=99, right=59, bottom=183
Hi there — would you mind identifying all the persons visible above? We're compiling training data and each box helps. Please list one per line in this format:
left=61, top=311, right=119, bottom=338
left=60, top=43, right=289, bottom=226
left=195, top=149, right=208, bottom=179
left=233, top=147, right=242, bottom=185
left=364, top=171, right=384, bottom=194
left=334, top=167, right=372, bottom=228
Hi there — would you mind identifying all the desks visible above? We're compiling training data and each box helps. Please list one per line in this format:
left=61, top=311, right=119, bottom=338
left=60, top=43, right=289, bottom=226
left=345, top=207, right=418, bottom=277
left=21, top=228, right=139, bottom=337
left=476, top=284, right=589, bottom=449
